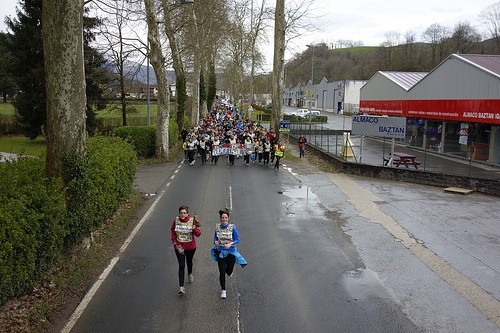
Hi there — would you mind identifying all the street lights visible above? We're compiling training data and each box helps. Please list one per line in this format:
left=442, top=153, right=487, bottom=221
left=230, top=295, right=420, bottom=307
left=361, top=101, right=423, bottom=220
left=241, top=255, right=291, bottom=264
left=306, top=44, right=321, bottom=84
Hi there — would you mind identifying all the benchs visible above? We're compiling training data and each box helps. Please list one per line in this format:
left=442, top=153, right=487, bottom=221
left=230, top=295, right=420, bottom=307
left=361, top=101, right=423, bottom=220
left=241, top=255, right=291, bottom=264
left=382, top=157, right=423, bottom=165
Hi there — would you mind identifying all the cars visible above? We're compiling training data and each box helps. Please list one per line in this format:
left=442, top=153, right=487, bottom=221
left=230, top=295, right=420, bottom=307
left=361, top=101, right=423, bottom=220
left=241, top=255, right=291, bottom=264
left=291, top=109, right=319, bottom=117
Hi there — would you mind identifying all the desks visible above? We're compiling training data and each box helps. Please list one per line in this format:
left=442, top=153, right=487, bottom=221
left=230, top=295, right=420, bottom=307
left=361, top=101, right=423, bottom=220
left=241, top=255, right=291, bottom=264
left=385, top=152, right=418, bottom=169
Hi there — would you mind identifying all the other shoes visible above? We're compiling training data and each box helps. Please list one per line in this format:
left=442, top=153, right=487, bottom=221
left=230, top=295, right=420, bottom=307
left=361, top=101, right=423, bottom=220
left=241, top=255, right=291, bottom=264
left=229, top=271, right=233, bottom=277
left=237, top=156, right=242, bottom=159
left=252, top=161, right=254, bottom=164
left=246, top=164, right=250, bottom=166
left=219, top=289, right=226, bottom=299
left=258, top=163, right=261, bottom=165
left=190, top=162, right=194, bottom=166
left=277, top=168, right=279, bottom=170
left=192, top=160, right=195, bottom=162
left=188, top=273, right=194, bottom=284
left=178, top=286, right=186, bottom=295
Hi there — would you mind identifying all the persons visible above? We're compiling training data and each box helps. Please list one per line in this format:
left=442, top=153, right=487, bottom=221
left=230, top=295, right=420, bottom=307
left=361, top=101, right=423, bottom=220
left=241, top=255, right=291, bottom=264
left=273, top=141, right=286, bottom=170
left=299, top=133, right=307, bottom=158
left=170, top=205, right=201, bottom=294
left=211, top=208, right=248, bottom=300
left=181, top=92, right=276, bottom=166
